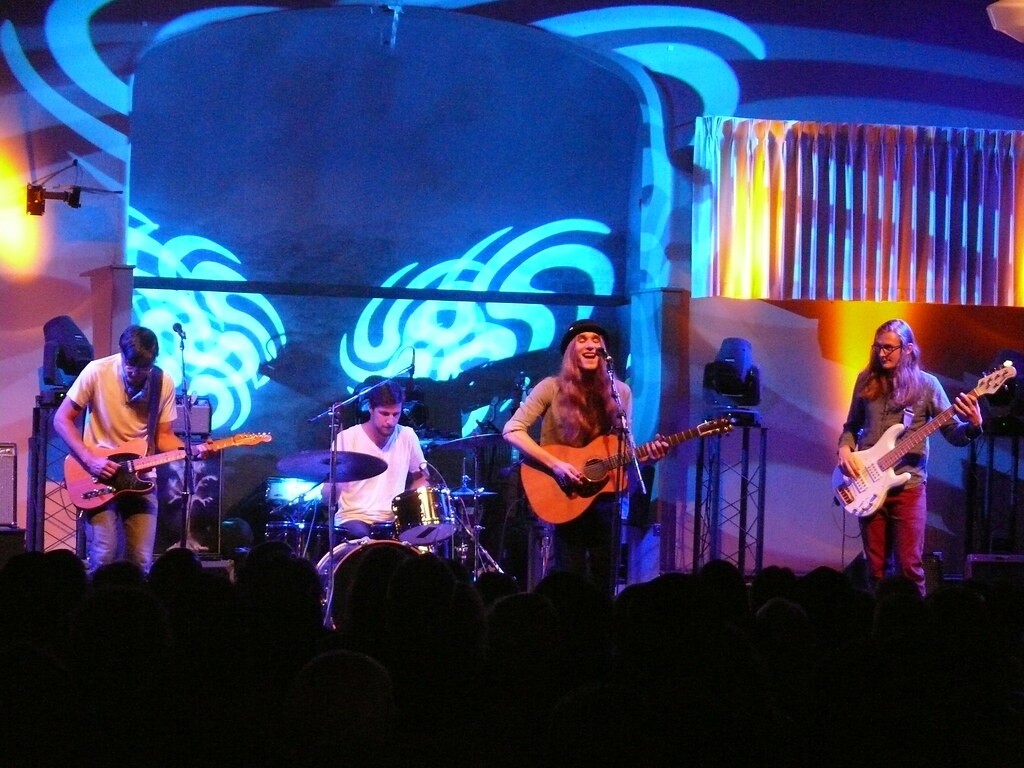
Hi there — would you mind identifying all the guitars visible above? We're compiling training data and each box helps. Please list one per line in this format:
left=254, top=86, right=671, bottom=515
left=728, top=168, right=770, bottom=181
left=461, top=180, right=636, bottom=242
left=65, top=428, right=272, bottom=511
left=518, top=417, right=737, bottom=527
left=831, top=363, right=1018, bottom=516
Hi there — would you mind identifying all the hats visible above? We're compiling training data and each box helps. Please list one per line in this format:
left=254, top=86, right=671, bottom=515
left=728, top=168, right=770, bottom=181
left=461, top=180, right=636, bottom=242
left=560, top=319, right=610, bottom=356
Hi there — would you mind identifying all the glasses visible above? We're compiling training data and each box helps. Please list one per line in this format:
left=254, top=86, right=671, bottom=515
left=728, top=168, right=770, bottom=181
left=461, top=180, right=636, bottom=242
left=123, top=357, right=154, bottom=373
left=871, top=344, right=902, bottom=354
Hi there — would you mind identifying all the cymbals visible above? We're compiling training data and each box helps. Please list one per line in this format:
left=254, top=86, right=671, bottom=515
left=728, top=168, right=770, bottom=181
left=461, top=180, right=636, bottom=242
left=448, top=488, right=500, bottom=500
left=276, top=451, right=389, bottom=485
left=430, top=433, right=507, bottom=454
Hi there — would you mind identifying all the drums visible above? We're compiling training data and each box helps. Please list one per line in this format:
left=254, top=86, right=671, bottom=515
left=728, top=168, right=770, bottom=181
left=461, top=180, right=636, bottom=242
left=313, top=535, right=423, bottom=624
left=392, top=483, right=460, bottom=547
left=264, top=522, right=351, bottom=566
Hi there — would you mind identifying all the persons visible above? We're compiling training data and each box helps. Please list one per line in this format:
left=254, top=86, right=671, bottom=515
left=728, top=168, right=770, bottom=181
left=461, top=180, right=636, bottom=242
left=502, top=320, right=669, bottom=599
left=53, top=325, right=214, bottom=573
left=838, top=320, right=983, bottom=597
left=320, top=380, right=429, bottom=539
left=0, top=542, right=1024, bottom=768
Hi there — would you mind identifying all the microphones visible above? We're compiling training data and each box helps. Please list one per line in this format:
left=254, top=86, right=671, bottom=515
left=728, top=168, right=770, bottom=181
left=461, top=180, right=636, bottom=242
left=172, top=323, right=186, bottom=340
left=419, top=467, right=437, bottom=484
left=405, top=348, right=416, bottom=403
left=595, top=347, right=613, bottom=362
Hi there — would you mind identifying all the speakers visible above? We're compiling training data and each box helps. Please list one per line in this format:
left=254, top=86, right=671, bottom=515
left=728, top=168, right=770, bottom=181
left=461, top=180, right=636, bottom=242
left=0, top=443, right=18, bottom=529
left=964, top=554, right=1024, bottom=584
left=151, top=394, right=224, bottom=559
